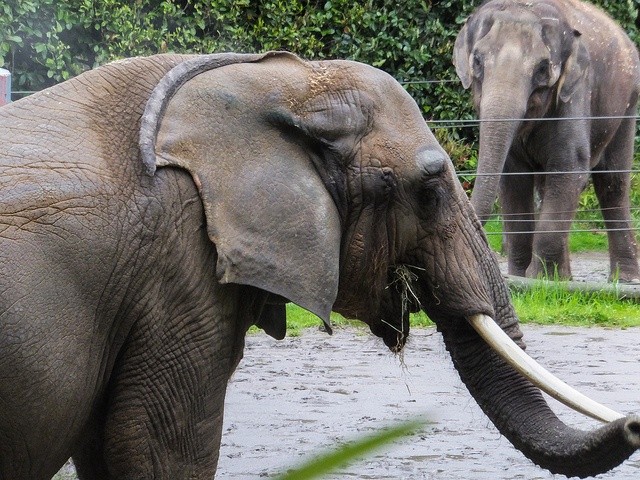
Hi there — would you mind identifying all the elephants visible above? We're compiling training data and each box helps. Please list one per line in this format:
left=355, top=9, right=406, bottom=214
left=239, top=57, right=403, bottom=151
left=451, top=0, right=640, bottom=286
left=0, top=49, right=640, bottom=480
